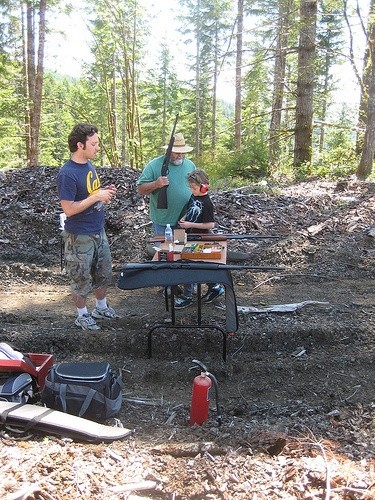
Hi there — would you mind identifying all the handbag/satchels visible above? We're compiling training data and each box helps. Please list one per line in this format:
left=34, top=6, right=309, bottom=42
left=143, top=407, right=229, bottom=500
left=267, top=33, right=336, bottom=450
left=0, top=401, right=133, bottom=443
left=44, top=362, right=123, bottom=422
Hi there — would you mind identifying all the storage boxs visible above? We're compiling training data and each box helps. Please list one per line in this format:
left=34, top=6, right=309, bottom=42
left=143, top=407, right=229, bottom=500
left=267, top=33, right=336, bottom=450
left=0, top=353, right=54, bottom=392
left=181, top=245, right=222, bottom=258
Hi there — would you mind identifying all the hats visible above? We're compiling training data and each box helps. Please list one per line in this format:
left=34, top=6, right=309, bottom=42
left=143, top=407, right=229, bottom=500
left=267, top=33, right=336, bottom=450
left=162, top=133, right=193, bottom=153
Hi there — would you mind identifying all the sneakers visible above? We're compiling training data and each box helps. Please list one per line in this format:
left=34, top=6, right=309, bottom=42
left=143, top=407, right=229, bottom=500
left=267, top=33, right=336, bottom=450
left=75, top=312, right=101, bottom=330
left=201, top=284, right=224, bottom=303
left=91, top=305, right=119, bottom=321
left=174, top=295, right=193, bottom=309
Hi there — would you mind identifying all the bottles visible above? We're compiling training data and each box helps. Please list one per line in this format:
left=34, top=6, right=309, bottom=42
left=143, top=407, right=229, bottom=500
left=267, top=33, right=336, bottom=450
left=95, top=200, right=106, bottom=212
left=165, top=224, right=172, bottom=247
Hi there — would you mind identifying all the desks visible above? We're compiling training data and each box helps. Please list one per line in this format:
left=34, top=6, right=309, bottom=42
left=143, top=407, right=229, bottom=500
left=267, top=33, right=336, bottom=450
left=149, top=240, right=227, bottom=360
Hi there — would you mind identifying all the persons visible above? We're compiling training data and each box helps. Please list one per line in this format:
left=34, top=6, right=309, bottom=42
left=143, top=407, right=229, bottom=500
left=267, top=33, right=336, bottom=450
left=137, top=132, right=197, bottom=298
left=56, top=123, right=121, bottom=331
left=174, top=168, right=224, bottom=310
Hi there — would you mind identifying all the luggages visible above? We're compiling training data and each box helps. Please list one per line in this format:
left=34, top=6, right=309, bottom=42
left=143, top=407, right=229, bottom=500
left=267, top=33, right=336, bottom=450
left=1, top=372, right=38, bottom=412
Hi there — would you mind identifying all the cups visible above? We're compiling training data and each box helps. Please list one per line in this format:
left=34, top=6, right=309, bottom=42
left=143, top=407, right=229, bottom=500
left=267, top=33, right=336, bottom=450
left=173, top=229, right=187, bottom=246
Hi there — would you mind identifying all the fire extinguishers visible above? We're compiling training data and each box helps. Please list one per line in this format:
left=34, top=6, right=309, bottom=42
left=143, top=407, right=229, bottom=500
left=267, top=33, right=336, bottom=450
left=189, top=360, right=221, bottom=426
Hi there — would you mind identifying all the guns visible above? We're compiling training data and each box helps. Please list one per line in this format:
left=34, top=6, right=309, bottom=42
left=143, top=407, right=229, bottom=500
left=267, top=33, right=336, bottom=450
left=156, top=113, right=179, bottom=210
left=146, top=233, right=280, bottom=244
left=122, top=261, right=286, bottom=270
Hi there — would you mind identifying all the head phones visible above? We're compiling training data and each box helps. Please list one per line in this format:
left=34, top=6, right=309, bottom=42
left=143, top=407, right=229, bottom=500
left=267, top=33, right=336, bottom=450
left=190, top=176, right=208, bottom=193
